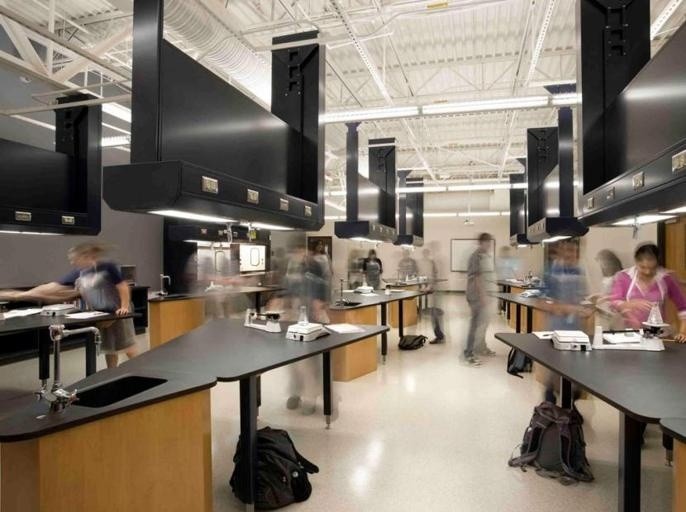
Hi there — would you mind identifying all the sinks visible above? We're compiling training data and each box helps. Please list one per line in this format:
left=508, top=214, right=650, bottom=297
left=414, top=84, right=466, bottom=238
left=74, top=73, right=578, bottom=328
left=164, top=294, right=192, bottom=299
left=335, top=300, right=362, bottom=307
left=69, top=373, right=168, bottom=408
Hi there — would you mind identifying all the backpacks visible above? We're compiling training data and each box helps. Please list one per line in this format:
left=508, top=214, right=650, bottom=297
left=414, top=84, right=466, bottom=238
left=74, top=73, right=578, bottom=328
left=229, top=426, right=319, bottom=511
left=399, top=334, right=427, bottom=349
left=507, top=347, right=532, bottom=378
left=509, top=401, right=594, bottom=485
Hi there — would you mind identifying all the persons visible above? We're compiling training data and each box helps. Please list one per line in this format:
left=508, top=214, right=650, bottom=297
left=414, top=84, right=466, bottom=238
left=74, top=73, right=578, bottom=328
left=267, top=231, right=686, bottom=420
left=16, top=244, right=137, bottom=369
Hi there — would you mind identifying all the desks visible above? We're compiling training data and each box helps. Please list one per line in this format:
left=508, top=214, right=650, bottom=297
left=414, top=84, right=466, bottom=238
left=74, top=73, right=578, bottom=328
left=659, top=414, right=686, bottom=512
left=324, top=282, right=434, bottom=380
left=146, top=284, right=289, bottom=348
left=378, top=274, right=449, bottom=316
left=490, top=287, right=597, bottom=404
left=494, top=277, right=540, bottom=316
left=494, top=330, right=685, bottom=512
left=0, top=308, right=144, bottom=392
left=0, top=316, right=394, bottom=509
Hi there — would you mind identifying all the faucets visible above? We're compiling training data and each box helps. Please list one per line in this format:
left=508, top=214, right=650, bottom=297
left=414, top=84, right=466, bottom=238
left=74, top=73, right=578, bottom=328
left=338, top=278, right=344, bottom=308
left=33, top=324, right=103, bottom=411
left=156, top=272, right=171, bottom=296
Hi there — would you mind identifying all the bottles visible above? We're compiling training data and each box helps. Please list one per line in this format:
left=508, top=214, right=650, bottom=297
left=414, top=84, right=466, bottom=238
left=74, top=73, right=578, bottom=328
left=298, top=305, right=309, bottom=325
left=592, top=322, right=604, bottom=346
